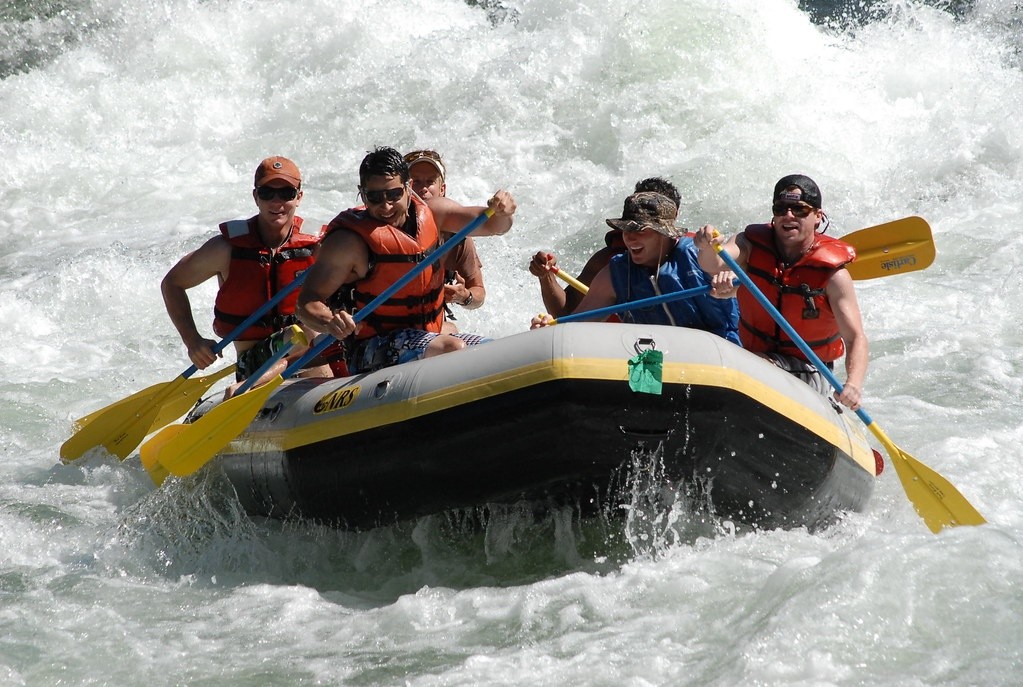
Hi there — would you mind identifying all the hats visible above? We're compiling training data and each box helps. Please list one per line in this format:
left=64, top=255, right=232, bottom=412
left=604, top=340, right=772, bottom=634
left=404, top=151, right=445, bottom=183
left=255, top=157, right=301, bottom=190
left=773, top=175, right=821, bottom=209
left=606, top=191, right=688, bottom=239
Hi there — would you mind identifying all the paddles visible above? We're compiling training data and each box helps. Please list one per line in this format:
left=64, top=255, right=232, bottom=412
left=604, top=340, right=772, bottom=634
left=539, top=253, right=590, bottom=296
left=707, top=233, right=987, bottom=535
left=532, top=215, right=935, bottom=328
left=141, top=323, right=306, bottom=487
left=70, top=360, right=237, bottom=437
left=159, top=201, right=497, bottom=476
left=60, top=263, right=315, bottom=470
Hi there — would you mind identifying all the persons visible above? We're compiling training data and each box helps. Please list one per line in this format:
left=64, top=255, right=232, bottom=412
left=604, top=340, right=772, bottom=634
left=160, top=156, right=334, bottom=402
left=404, top=150, right=486, bottom=334
left=693, top=174, right=869, bottom=411
left=294, top=148, right=517, bottom=376
left=529, top=179, right=697, bottom=323
left=529, top=191, right=740, bottom=330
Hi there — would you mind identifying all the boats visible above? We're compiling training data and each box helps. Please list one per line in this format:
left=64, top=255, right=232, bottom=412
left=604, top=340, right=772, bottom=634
left=180, top=323, right=876, bottom=557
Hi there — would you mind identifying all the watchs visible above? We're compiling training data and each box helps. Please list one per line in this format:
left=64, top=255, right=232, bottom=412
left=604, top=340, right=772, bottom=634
left=456, top=292, right=473, bottom=307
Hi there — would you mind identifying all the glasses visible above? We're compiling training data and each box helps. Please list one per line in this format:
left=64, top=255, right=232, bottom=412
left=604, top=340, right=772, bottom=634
left=361, top=185, right=406, bottom=206
left=773, top=201, right=816, bottom=218
left=257, top=185, right=297, bottom=202
